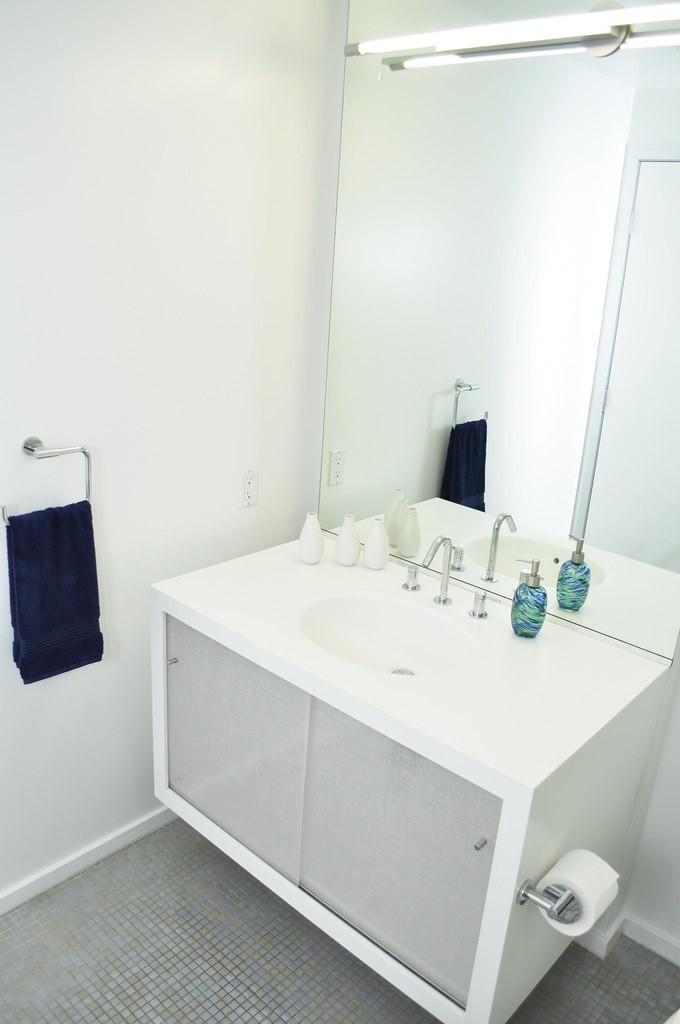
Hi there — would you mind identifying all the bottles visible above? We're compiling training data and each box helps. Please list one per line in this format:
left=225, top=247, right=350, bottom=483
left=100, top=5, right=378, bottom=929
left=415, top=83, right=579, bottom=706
left=335, top=515, right=360, bottom=565
left=384, top=488, right=420, bottom=557
left=364, top=518, right=389, bottom=569
left=297, top=512, right=323, bottom=565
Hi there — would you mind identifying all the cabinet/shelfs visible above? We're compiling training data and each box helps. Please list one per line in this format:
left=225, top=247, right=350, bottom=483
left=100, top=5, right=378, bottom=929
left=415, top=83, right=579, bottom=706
left=163, top=613, right=505, bottom=1011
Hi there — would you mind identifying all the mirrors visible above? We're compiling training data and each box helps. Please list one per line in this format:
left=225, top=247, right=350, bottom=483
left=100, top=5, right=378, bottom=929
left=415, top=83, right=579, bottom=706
left=318, top=0, right=680, bottom=664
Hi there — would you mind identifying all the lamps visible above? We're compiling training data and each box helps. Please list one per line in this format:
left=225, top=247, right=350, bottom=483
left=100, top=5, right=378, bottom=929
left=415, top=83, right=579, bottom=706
left=344, top=3, right=680, bottom=72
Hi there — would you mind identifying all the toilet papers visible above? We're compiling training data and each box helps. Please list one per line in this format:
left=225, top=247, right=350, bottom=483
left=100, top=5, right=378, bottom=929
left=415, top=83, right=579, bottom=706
left=537, top=849, right=622, bottom=938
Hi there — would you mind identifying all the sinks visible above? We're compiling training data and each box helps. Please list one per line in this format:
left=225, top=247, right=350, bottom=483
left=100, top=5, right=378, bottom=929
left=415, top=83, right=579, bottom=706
left=465, top=533, right=607, bottom=591
left=298, top=591, right=495, bottom=683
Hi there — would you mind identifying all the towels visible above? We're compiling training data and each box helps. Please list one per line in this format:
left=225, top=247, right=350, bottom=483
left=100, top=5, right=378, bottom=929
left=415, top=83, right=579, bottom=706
left=440, top=418, right=487, bottom=512
left=4, top=498, right=106, bottom=686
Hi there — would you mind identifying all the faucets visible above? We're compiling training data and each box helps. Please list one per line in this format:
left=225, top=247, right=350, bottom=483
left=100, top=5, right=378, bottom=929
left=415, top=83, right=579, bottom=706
left=421, top=535, right=453, bottom=606
left=482, top=512, right=518, bottom=583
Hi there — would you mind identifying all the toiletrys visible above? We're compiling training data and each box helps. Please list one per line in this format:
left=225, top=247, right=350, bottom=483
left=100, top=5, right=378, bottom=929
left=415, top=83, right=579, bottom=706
left=556, top=531, right=591, bottom=612
left=509, top=558, right=550, bottom=638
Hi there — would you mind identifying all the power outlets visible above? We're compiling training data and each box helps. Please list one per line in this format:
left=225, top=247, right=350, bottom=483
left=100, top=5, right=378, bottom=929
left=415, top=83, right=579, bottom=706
left=328, top=450, right=345, bottom=486
left=244, top=469, right=259, bottom=508
